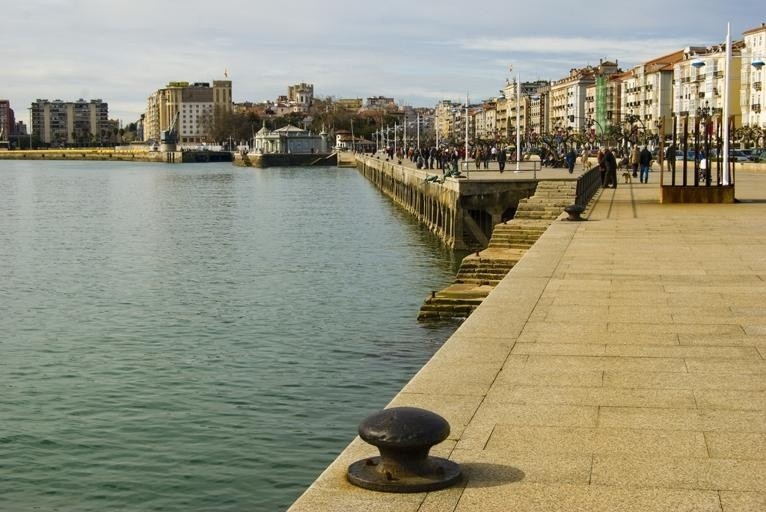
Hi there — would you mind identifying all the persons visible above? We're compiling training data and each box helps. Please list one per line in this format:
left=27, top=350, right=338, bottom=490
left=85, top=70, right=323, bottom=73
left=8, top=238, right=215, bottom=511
left=639, top=145, right=652, bottom=183
left=384, top=136, right=620, bottom=190
left=629, top=143, right=641, bottom=177
left=618, top=154, right=629, bottom=171
left=666, top=143, right=675, bottom=172
left=699, top=154, right=711, bottom=182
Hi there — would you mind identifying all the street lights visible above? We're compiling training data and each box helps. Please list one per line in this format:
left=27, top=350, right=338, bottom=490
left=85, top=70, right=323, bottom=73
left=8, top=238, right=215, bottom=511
left=690, top=20, right=766, bottom=203
left=498, top=71, right=543, bottom=174
left=371, top=107, right=443, bottom=170
left=448, top=93, right=486, bottom=168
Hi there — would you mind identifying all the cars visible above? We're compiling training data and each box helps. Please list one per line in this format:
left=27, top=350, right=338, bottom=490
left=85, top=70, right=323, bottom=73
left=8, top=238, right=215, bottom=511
left=581, top=139, right=766, bottom=164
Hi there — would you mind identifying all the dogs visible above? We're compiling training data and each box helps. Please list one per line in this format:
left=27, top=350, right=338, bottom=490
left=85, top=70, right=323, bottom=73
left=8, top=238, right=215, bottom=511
left=621, top=173, right=632, bottom=184
left=585, top=161, right=592, bottom=171
